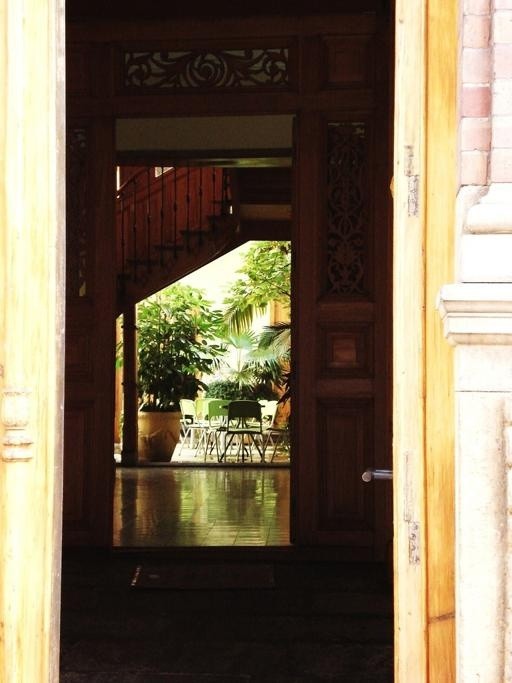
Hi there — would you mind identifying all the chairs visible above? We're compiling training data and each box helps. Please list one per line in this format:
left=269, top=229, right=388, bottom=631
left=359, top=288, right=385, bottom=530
left=178, top=398, right=290, bottom=463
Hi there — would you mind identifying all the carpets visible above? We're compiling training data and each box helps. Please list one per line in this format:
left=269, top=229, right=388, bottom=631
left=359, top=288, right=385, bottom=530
left=130, top=565, right=277, bottom=590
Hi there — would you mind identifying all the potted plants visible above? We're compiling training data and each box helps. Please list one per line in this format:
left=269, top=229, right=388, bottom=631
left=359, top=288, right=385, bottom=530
left=116, top=283, right=229, bottom=462
